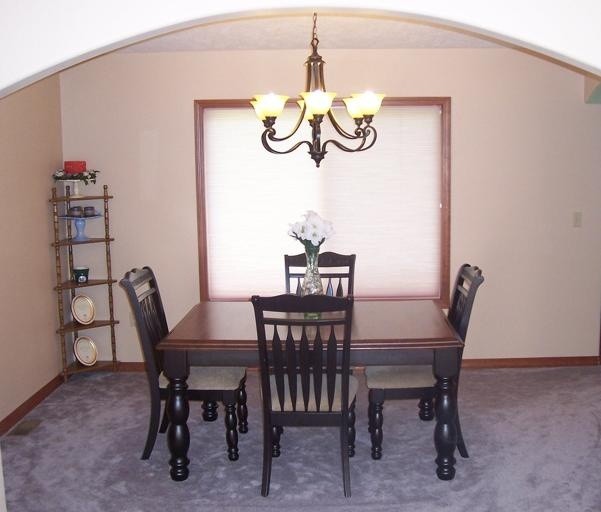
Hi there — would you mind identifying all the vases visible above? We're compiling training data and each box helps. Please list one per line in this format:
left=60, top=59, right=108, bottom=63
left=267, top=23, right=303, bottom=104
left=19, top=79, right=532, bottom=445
left=300, top=249, right=323, bottom=320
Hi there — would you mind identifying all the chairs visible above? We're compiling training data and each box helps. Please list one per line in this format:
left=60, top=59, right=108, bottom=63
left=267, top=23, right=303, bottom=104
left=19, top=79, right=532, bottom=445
left=257, top=252, right=357, bottom=397
left=250, top=293, right=360, bottom=498
left=364, top=263, right=485, bottom=460
left=118, top=265, right=248, bottom=460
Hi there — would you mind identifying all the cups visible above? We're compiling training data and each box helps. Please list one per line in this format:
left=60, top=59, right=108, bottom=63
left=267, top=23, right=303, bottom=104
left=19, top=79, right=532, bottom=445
left=64, top=160, right=87, bottom=172
left=72, top=267, right=90, bottom=282
left=66, top=205, right=96, bottom=216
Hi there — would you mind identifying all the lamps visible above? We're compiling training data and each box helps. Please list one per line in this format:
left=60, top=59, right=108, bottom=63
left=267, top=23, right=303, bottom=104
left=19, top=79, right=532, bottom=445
left=249, top=12, right=386, bottom=168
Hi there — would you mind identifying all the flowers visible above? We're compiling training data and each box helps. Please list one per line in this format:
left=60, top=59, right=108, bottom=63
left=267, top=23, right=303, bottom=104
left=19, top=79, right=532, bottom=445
left=284, top=209, right=343, bottom=262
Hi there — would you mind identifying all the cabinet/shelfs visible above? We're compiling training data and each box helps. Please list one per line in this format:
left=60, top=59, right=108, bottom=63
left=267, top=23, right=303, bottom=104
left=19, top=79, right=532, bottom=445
left=48, top=184, right=120, bottom=383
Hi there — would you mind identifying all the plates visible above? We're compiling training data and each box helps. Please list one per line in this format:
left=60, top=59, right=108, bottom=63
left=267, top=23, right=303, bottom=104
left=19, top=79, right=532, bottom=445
left=73, top=334, right=100, bottom=367
left=71, top=293, right=96, bottom=325
